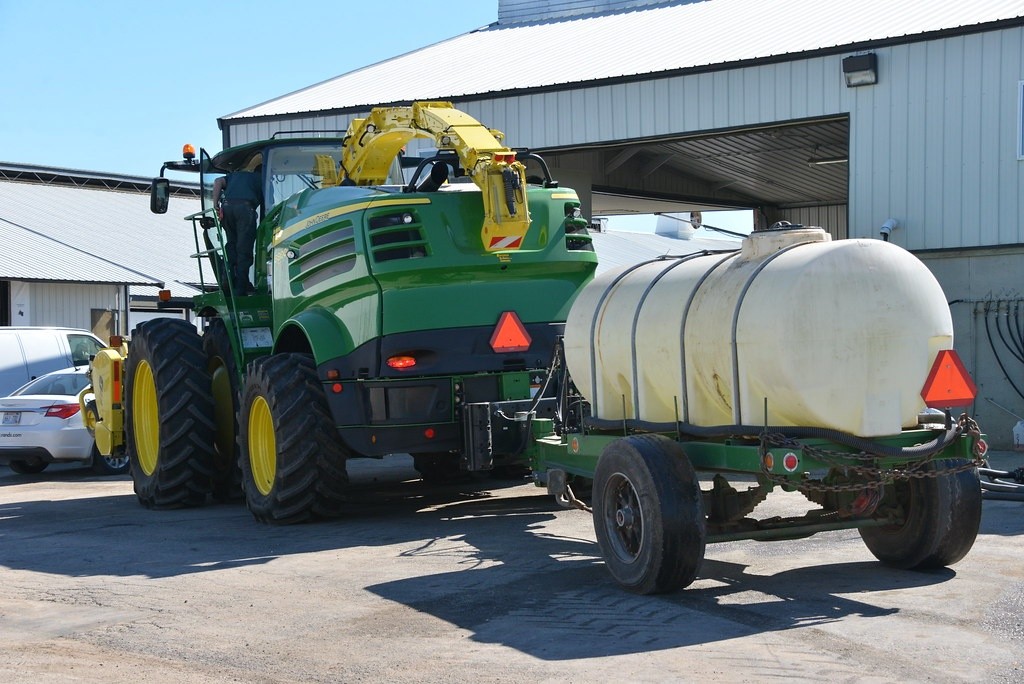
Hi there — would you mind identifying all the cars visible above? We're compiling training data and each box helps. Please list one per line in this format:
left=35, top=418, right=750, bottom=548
left=0, top=365, right=130, bottom=475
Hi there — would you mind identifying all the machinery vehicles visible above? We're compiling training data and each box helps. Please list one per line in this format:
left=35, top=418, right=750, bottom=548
left=79, top=101, right=990, bottom=597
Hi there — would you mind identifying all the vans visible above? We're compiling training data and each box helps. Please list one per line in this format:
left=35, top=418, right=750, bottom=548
left=0, top=326, right=116, bottom=399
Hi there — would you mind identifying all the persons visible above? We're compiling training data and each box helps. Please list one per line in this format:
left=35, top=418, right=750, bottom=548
left=213, top=164, right=264, bottom=298
left=73, top=342, right=90, bottom=362
